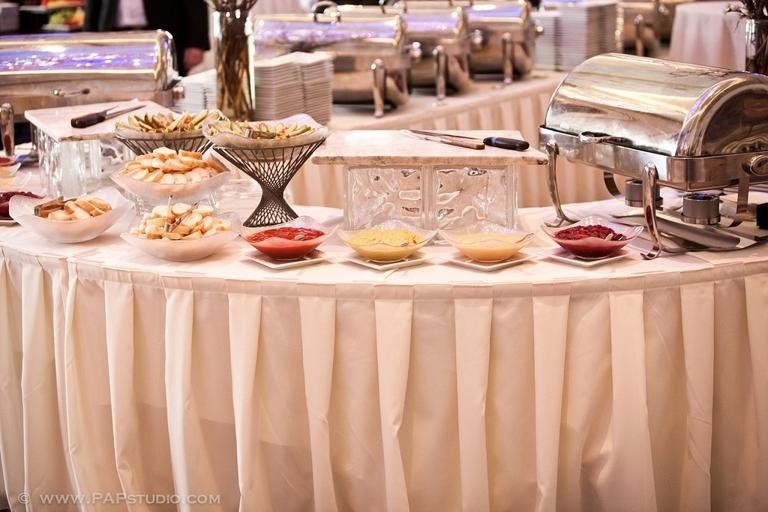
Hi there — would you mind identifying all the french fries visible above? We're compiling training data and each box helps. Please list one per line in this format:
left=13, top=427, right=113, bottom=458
left=207, top=120, right=314, bottom=141
left=116, top=109, right=222, bottom=133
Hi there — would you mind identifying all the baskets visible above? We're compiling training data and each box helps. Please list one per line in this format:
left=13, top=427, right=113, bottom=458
left=116, top=136, right=213, bottom=155
left=213, top=139, right=326, bottom=227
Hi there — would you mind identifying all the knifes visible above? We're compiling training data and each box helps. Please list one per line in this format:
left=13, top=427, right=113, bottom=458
left=70, top=102, right=148, bottom=129
left=407, top=120, right=529, bottom=148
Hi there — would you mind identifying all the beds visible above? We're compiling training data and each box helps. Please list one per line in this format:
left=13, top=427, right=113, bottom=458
left=0, top=72, right=768, bottom=512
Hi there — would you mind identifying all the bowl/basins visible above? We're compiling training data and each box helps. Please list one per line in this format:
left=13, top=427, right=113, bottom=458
left=335, top=218, right=437, bottom=262
left=7, top=193, right=129, bottom=245
left=437, top=218, right=535, bottom=262
left=201, top=112, right=326, bottom=160
left=539, top=213, right=645, bottom=259
left=235, top=215, right=336, bottom=259
left=109, top=165, right=238, bottom=200
left=115, top=122, right=203, bottom=149
left=122, top=226, right=238, bottom=264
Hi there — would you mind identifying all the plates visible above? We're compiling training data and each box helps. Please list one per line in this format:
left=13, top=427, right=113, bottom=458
left=541, top=241, right=631, bottom=269
left=254, top=50, right=335, bottom=126
left=342, top=249, right=434, bottom=274
left=242, top=249, right=329, bottom=272
left=180, top=67, right=219, bottom=110
left=445, top=250, right=532, bottom=273
left=531, top=4, right=625, bottom=73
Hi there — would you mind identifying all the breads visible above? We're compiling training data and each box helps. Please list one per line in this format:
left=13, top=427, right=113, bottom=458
left=130, top=203, right=232, bottom=240
left=36, top=197, right=110, bottom=222
left=124, top=147, right=228, bottom=179
